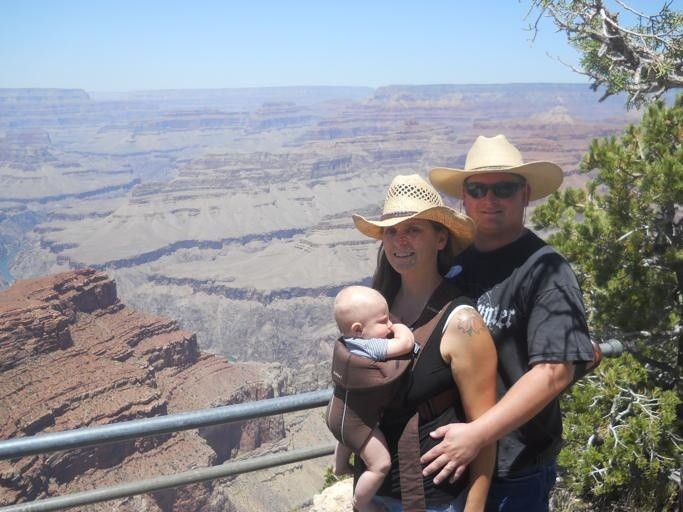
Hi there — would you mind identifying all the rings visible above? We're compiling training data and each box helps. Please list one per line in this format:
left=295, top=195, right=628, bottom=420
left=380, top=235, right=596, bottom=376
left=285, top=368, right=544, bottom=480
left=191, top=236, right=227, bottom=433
left=446, top=464, right=454, bottom=471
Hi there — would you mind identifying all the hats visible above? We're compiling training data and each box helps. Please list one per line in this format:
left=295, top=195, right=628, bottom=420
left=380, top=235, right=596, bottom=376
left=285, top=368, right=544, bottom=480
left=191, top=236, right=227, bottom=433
left=428, top=134, right=564, bottom=201
left=352, top=174, right=476, bottom=240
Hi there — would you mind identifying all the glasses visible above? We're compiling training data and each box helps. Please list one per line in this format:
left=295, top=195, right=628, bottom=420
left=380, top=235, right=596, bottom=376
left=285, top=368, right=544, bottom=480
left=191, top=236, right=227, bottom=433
left=462, top=181, right=525, bottom=199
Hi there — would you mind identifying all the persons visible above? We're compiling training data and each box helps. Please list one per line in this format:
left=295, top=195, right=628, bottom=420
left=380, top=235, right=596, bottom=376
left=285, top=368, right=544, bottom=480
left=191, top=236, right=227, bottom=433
left=419, top=132, right=596, bottom=512
left=351, top=172, right=500, bottom=512
left=323, top=285, right=416, bottom=511
